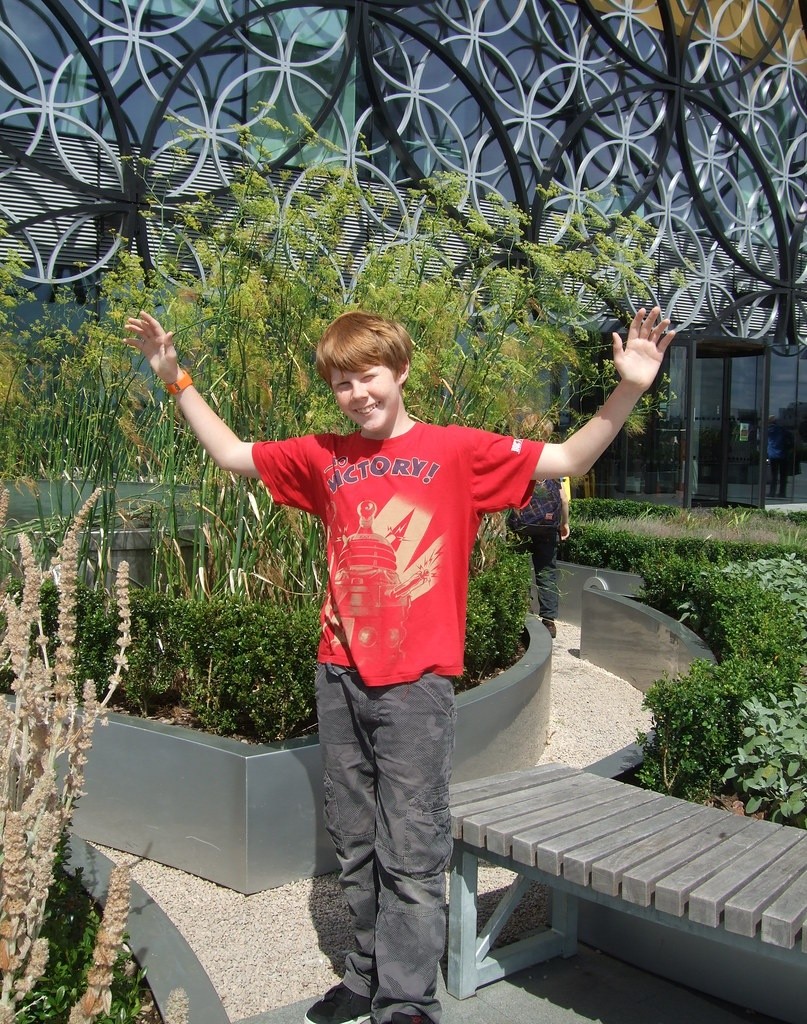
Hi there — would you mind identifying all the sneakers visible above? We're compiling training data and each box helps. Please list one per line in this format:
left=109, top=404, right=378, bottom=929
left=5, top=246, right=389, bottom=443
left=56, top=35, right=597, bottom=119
left=304, top=981, right=373, bottom=1024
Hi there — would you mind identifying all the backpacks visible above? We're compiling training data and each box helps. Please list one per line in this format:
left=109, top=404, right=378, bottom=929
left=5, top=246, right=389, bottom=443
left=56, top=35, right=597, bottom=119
left=505, top=479, right=562, bottom=557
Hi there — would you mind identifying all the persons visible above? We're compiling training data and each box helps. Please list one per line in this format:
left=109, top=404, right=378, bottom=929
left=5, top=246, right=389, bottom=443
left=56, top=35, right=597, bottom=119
left=739, top=414, right=791, bottom=498
left=503, top=413, right=571, bottom=638
left=119, top=307, right=678, bottom=1024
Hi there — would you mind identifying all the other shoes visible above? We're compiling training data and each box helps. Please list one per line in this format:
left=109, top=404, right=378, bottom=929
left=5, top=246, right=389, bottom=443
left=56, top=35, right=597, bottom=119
left=542, top=619, right=556, bottom=637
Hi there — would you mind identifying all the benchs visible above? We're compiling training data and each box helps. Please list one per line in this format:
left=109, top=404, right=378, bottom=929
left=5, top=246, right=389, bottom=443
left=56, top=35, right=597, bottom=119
left=445, top=763, right=807, bottom=1001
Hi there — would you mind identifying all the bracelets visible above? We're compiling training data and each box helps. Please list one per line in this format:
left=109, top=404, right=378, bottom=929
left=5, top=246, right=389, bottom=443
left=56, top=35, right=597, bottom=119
left=166, top=369, right=193, bottom=396
